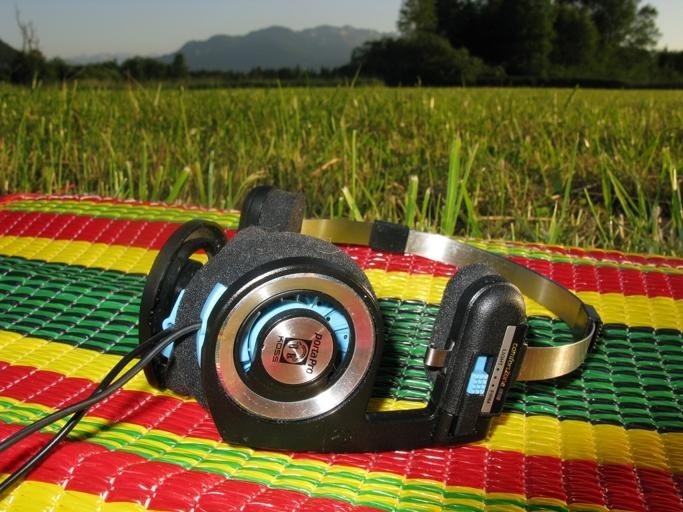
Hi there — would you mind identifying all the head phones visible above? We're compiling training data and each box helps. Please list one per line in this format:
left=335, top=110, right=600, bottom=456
left=137, top=183, right=605, bottom=451
